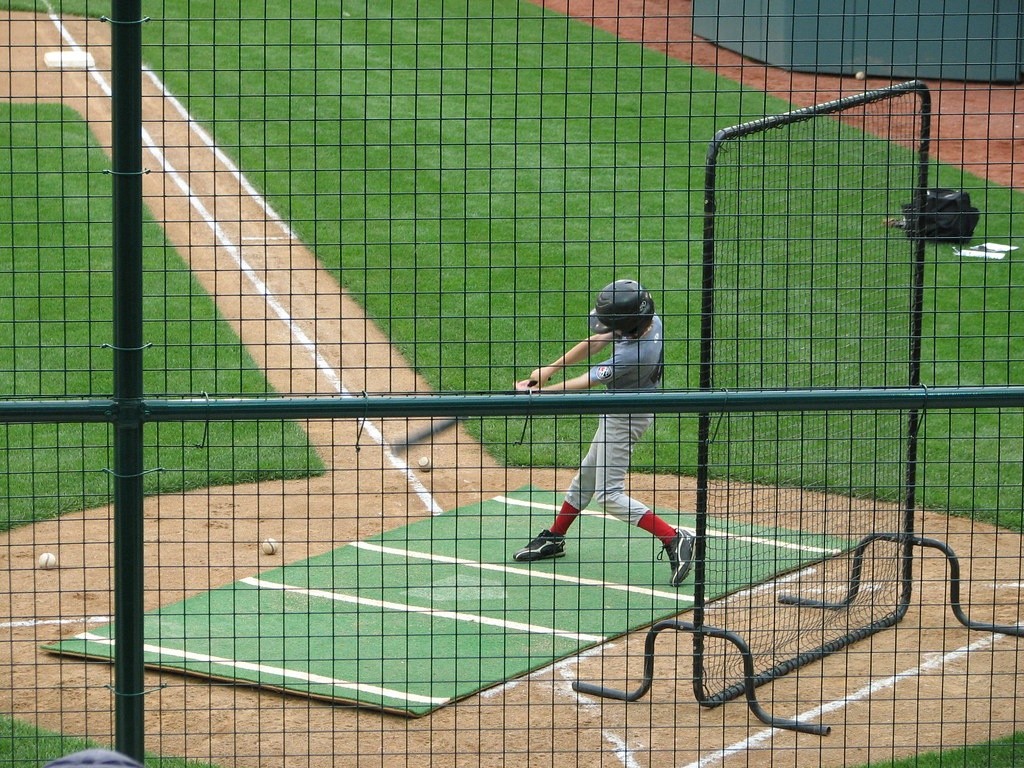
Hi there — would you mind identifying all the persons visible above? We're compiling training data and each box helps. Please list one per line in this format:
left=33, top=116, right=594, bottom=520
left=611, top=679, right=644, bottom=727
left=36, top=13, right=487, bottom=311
left=510, top=279, right=698, bottom=586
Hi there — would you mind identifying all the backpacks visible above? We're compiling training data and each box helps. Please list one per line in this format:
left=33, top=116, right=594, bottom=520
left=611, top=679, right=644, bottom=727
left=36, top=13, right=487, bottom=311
left=904, top=188, right=980, bottom=243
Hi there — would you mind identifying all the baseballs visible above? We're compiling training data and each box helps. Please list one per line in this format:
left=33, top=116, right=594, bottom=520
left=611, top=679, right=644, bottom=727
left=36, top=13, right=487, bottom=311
left=38, top=552, right=57, bottom=570
left=418, top=457, right=431, bottom=472
left=262, top=538, right=278, bottom=555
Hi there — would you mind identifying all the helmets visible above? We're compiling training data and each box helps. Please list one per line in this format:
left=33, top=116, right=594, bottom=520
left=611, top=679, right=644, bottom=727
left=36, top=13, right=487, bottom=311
left=586, top=279, right=654, bottom=335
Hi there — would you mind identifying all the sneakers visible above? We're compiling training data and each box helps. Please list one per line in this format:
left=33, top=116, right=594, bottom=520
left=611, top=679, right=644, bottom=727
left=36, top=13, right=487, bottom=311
left=657, top=528, right=696, bottom=586
left=512, top=529, right=567, bottom=562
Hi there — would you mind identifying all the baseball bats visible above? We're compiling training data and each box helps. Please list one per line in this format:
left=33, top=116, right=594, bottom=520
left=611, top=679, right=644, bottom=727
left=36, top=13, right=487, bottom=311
left=391, top=374, right=551, bottom=454
